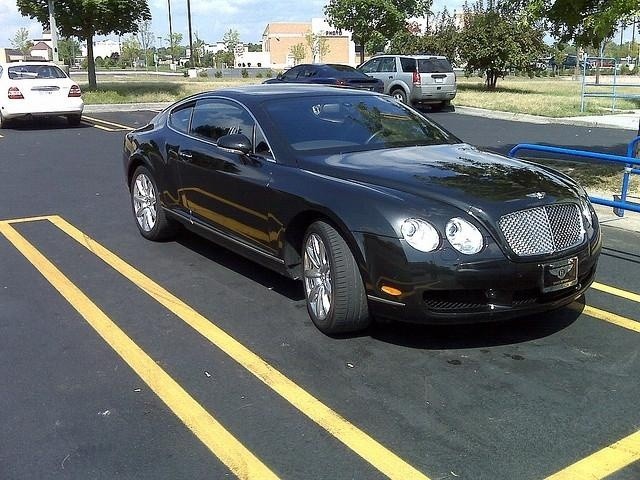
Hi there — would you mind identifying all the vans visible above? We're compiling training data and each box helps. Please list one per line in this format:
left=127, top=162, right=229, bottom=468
left=548, top=55, right=592, bottom=71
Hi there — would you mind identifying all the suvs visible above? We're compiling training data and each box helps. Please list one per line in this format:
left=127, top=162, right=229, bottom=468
left=356, top=53, right=457, bottom=108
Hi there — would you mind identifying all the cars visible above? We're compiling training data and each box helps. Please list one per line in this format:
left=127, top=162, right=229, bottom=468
left=0, top=61, right=84, bottom=129
left=121, top=82, right=601, bottom=329
left=261, top=62, right=385, bottom=93
